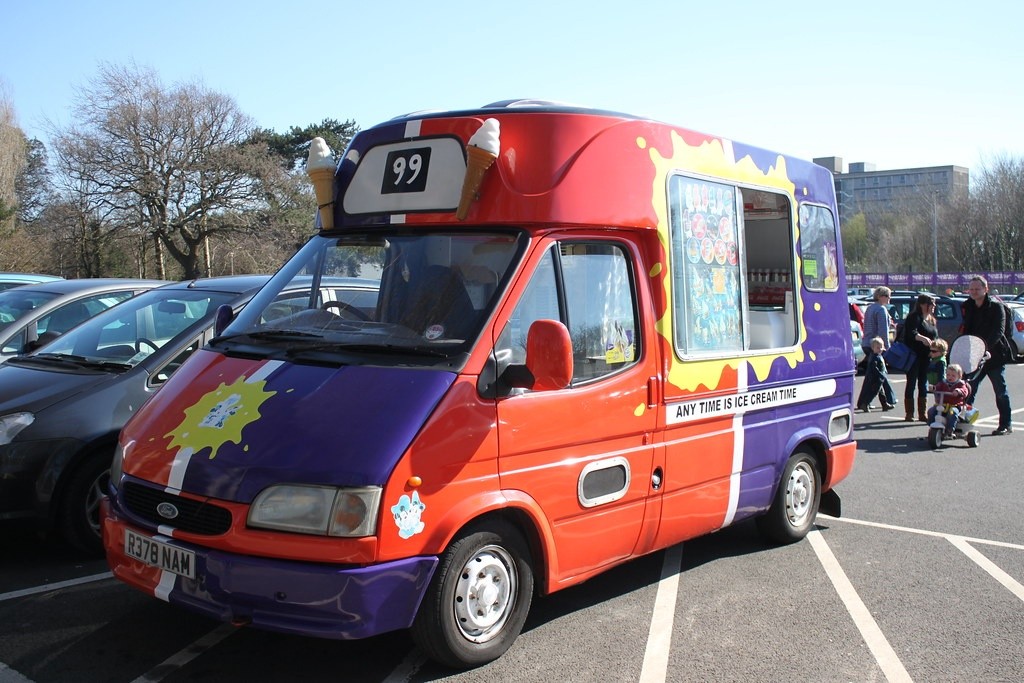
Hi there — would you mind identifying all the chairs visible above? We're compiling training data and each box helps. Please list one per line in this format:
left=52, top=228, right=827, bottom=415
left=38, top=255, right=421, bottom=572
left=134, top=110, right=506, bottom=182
left=47, top=303, right=92, bottom=334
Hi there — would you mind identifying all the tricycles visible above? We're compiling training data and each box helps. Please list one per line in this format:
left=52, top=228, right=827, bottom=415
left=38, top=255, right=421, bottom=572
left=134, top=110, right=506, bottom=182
left=925, top=335, right=987, bottom=451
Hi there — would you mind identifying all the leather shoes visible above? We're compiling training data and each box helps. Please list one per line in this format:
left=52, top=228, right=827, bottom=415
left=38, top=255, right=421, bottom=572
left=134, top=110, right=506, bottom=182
left=992, top=425, right=1012, bottom=436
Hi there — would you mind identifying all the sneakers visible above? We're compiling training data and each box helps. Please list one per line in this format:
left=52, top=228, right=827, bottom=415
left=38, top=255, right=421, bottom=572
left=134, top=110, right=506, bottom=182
left=882, top=402, right=895, bottom=411
left=944, top=429, right=953, bottom=440
left=858, top=404, right=869, bottom=412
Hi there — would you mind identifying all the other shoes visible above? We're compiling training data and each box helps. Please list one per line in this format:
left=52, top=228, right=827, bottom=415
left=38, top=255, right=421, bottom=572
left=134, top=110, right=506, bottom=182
left=870, top=405, right=875, bottom=409
left=895, top=399, right=898, bottom=403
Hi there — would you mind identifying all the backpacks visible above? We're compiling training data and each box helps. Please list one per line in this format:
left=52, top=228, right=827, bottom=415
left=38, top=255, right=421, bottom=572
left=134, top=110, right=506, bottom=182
left=999, top=304, right=1019, bottom=361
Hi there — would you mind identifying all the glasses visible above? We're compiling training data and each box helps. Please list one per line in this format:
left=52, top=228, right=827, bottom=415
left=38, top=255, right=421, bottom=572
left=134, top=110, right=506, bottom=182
left=882, top=294, right=889, bottom=297
left=929, top=350, right=940, bottom=353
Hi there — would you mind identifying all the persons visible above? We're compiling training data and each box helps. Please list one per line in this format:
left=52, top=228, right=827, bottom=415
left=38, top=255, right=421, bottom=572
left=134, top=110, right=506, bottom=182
left=902, top=275, right=1015, bottom=436
left=857, top=286, right=899, bottom=413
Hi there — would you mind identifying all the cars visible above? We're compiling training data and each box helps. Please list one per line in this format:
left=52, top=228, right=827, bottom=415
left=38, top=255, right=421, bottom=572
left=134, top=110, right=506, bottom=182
left=0, top=272, right=197, bottom=350
left=0, top=273, right=383, bottom=560
left=847, top=286, right=1023, bottom=371
left=1, top=277, right=265, bottom=375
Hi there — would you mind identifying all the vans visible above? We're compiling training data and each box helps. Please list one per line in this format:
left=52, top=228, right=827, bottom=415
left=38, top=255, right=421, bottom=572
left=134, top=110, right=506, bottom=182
left=91, top=97, right=860, bottom=670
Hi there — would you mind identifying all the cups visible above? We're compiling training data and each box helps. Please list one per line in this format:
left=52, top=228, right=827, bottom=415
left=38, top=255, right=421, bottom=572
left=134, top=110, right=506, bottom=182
left=762, top=269, right=770, bottom=282
left=787, top=270, right=791, bottom=282
left=756, top=269, right=762, bottom=282
left=771, top=268, right=779, bottom=282
left=749, top=269, right=756, bottom=281
left=781, top=269, right=787, bottom=283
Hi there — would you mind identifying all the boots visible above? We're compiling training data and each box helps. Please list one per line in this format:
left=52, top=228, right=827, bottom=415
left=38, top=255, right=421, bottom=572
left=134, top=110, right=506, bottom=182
left=918, top=399, right=928, bottom=422
left=904, top=399, right=915, bottom=422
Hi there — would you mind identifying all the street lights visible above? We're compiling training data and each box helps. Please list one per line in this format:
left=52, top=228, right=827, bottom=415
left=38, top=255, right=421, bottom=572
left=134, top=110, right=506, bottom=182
left=930, top=187, right=943, bottom=272
